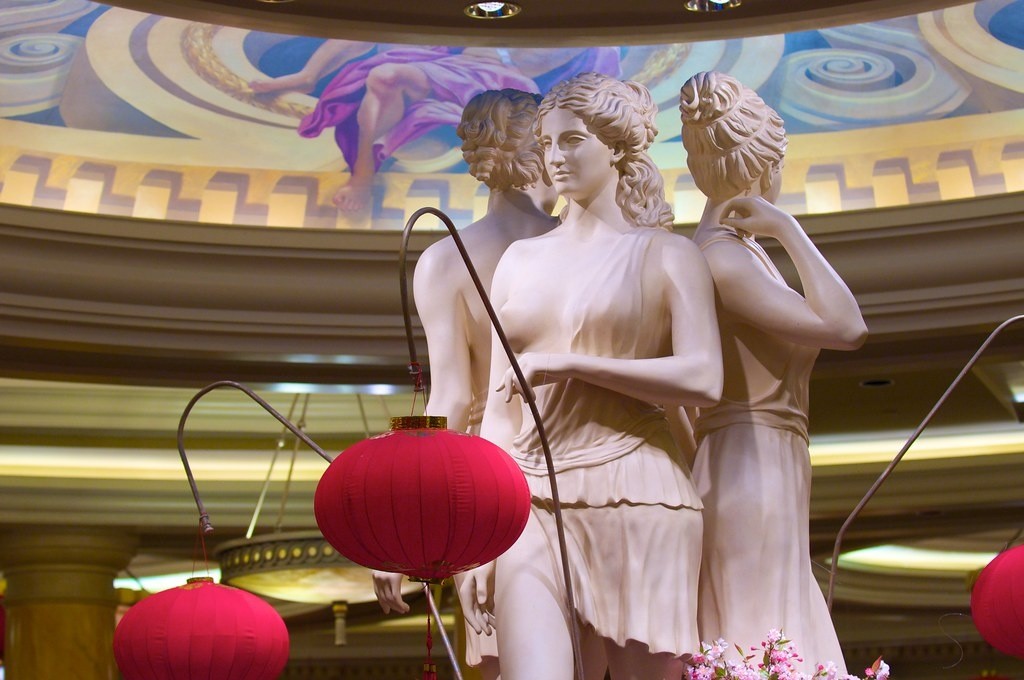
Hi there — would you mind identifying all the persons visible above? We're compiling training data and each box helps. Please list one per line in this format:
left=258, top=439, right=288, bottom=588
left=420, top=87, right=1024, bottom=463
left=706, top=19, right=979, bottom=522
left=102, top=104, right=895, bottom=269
left=372, top=71, right=869, bottom=680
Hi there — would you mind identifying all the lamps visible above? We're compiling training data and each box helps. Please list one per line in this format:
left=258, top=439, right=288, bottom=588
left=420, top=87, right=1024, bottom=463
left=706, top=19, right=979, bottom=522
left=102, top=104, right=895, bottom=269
left=211, top=389, right=427, bottom=648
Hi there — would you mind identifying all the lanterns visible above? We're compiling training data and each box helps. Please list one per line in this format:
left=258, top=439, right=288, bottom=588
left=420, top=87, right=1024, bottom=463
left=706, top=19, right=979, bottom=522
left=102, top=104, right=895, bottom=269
left=969, top=544, right=1024, bottom=658
left=315, top=415, right=531, bottom=680
left=113, top=577, right=290, bottom=680
